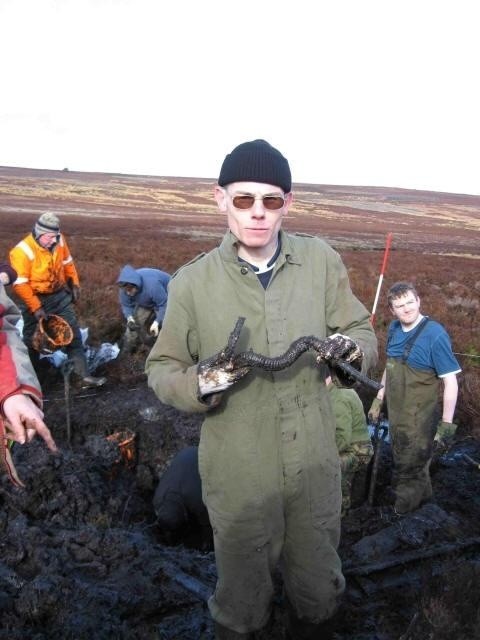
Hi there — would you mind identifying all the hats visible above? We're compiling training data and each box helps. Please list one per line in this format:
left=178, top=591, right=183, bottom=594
left=35, top=212, right=61, bottom=240
left=218, top=139, right=291, bottom=193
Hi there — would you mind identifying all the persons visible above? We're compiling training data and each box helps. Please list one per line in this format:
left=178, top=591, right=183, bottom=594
left=0, top=260, right=18, bottom=286
left=116, top=263, right=174, bottom=356
left=326, top=368, right=374, bottom=520
left=141, top=138, right=379, bottom=640
left=10, top=211, right=108, bottom=392
left=151, top=447, right=214, bottom=552
left=0, top=278, right=60, bottom=489
left=367, top=281, right=463, bottom=524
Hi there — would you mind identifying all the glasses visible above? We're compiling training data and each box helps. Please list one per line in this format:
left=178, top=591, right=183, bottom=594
left=233, top=195, right=285, bottom=210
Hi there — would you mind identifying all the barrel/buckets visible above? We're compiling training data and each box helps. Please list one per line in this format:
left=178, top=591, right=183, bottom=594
left=107, top=429, right=137, bottom=469
left=31, top=312, right=74, bottom=358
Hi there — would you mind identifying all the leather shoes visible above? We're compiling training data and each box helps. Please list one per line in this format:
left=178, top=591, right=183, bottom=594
left=69, top=375, right=107, bottom=390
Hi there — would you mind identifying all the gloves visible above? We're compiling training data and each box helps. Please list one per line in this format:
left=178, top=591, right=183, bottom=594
left=71, top=286, right=81, bottom=304
left=126, top=315, right=136, bottom=324
left=35, top=308, right=48, bottom=322
left=316, top=333, right=362, bottom=365
left=150, top=320, right=159, bottom=337
left=433, top=421, right=459, bottom=455
left=367, top=398, right=384, bottom=425
left=198, top=347, right=250, bottom=398
